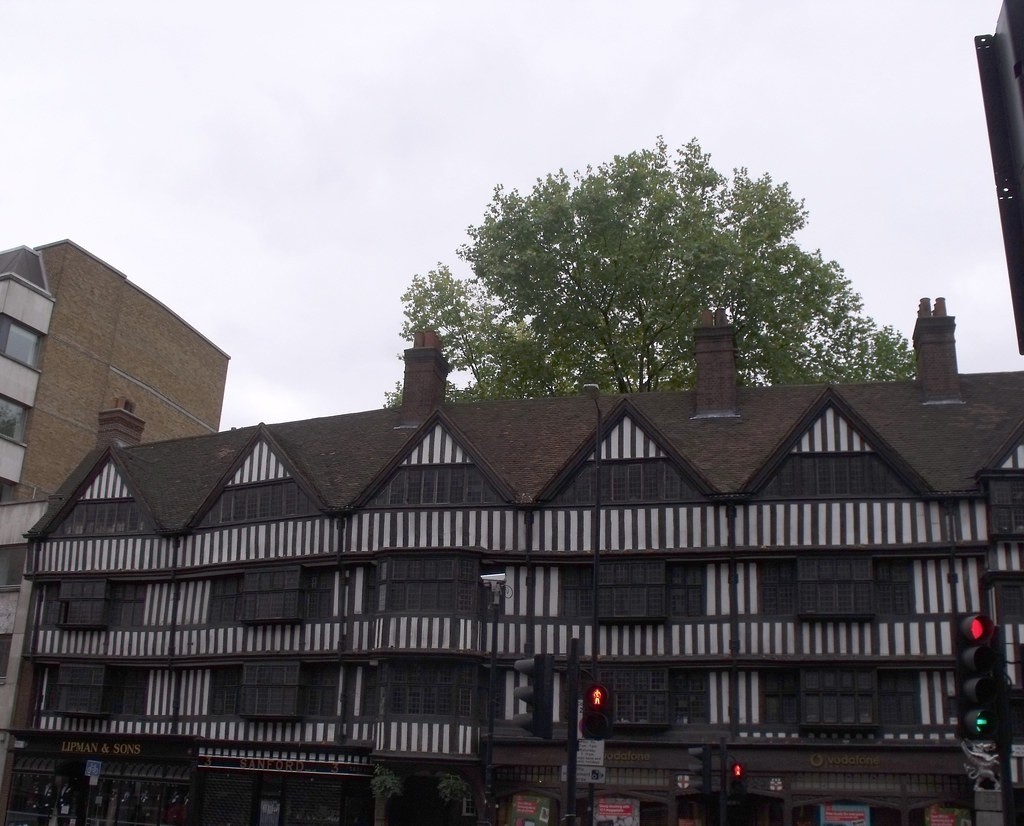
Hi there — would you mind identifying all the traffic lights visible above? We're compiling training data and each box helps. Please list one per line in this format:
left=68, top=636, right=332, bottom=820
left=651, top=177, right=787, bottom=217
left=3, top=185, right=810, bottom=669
left=950, top=610, right=1007, bottom=742
left=727, top=760, right=749, bottom=798
left=686, top=744, right=712, bottom=797
left=511, top=652, right=555, bottom=739
left=580, top=680, right=615, bottom=741
